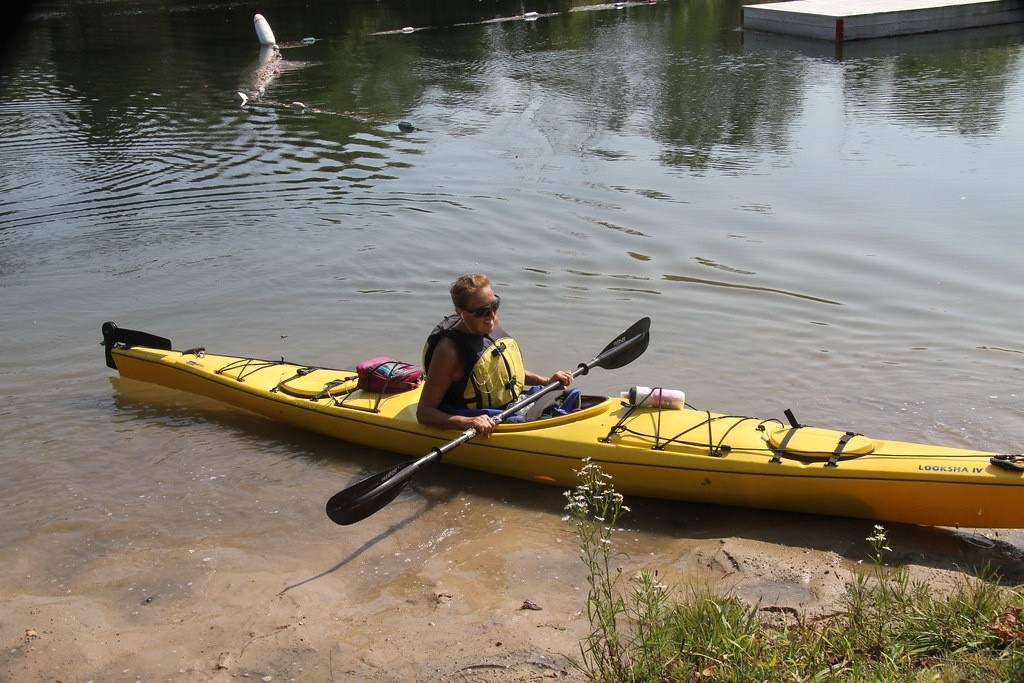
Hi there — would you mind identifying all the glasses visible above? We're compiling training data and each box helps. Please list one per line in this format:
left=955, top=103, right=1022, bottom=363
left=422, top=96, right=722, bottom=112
left=460, top=293, right=501, bottom=318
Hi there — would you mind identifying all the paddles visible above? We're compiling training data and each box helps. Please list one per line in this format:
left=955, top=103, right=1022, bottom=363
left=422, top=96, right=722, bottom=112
left=322, top=316, right=656, bottom=526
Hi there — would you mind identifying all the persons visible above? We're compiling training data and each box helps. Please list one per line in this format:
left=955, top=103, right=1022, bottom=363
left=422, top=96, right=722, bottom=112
left=416, top=274, right=573, bottom=440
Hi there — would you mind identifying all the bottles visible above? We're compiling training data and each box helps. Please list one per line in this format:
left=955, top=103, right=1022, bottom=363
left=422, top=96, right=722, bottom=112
left=619, top=386, right=685, bottom=410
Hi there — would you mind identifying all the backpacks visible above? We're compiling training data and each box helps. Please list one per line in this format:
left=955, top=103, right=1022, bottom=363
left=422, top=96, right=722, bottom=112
left=356, top=356, right=424, bottom=393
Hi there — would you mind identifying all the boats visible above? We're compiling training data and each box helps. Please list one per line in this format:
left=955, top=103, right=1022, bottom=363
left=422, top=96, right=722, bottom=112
left=97, top=317, right=1024, bottom=532
